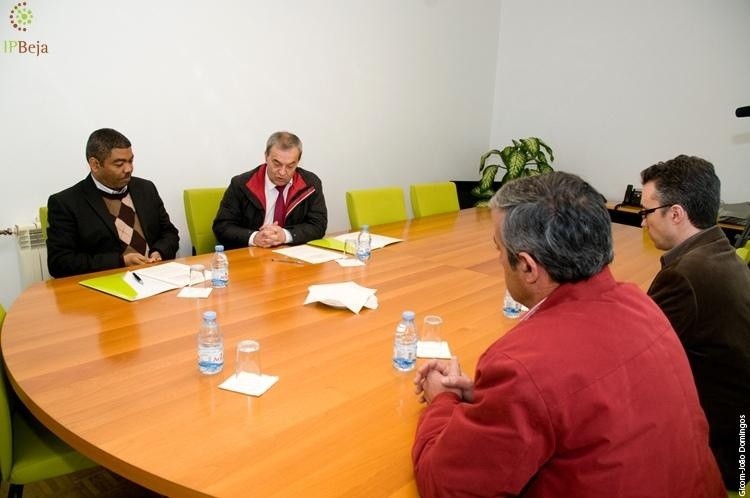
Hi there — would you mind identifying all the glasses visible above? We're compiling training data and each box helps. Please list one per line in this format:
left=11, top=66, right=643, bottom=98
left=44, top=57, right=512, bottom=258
left=638, top=203, right=673, bottom=219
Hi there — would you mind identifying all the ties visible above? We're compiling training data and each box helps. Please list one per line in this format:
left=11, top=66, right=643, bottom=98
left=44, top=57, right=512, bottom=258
left=273, top=184, right=286, bottom=227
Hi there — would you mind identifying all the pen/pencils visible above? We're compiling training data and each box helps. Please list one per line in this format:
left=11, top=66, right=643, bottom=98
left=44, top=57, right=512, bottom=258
left=272, top=259, right=302, bottom=264
left=132, top=272, right=144, bottom=285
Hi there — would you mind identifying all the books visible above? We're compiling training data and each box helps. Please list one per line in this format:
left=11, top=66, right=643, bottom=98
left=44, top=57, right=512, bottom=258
left=306, top=230, right=404, bottom=254
left=78, top=260, right=217, bottom=301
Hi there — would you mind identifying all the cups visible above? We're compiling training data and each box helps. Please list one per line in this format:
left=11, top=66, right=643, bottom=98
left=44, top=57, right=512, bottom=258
left=189, top=264, right=206, bottom=293
left=420, top=315, right=443, bottom=354
left=235, top=339, right=262, bottom=385
left=343, top=238, right=358, bottom=262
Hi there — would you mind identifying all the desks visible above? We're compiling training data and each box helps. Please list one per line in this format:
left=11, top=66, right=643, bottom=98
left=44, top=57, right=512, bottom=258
left=0, top=205, right=671, bottom=496
left=603, top=200, right=750, bottom=248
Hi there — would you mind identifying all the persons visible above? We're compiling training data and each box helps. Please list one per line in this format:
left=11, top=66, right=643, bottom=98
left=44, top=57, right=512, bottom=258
left=638, top=154, right=749, bottom=488
left=46, top=127, right=181, bottom=279
left=213, top=130, right=328, bottom=252
left=412, top=171, right=730, bottom=497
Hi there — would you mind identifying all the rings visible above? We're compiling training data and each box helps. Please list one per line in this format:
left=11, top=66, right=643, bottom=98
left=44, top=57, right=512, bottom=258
left=274, top=227, right=277, bottom=232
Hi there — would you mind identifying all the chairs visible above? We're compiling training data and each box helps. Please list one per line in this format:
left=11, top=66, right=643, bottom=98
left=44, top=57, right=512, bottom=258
left=183, top=187, right=234, bottom=254
left=735, top=240, right=750, bottom=264
left=1, top=301, right=103, bottom=496
left=345, top=181, right=462, bottom=228
left=34, top=202, right=51, bottom=238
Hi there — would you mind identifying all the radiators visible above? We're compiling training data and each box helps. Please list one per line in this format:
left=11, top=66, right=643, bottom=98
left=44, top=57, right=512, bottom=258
left=0, top=219, right=54, bottom=292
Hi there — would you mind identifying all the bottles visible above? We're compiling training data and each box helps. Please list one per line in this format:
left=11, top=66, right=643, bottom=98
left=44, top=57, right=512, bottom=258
left=503, top=287, right=525, bottom=319
left=210, top=244, right=228, bottom=289
left=391, top=310, right=418, bottom=371
left=356, top=225, right=372, bottom=263
left=198, top=312, right=223, bottom=375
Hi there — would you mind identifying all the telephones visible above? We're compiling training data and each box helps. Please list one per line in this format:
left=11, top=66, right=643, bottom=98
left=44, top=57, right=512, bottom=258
left=624, top=185, right=642, bottom=207
